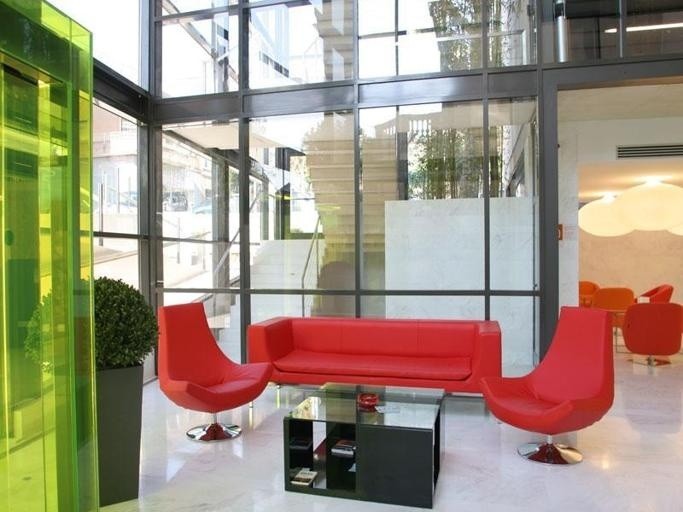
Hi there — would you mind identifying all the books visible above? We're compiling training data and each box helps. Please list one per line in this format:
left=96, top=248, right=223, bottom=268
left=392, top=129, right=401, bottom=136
left=331, top=440, right=356, bottom=456
left=290, top=469, right=318, bottom=487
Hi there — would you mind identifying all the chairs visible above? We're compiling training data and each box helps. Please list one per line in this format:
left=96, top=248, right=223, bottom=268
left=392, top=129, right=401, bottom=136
left=479, top=274, right=683, bottom=468
left=155, top=301, right=274, bottom=440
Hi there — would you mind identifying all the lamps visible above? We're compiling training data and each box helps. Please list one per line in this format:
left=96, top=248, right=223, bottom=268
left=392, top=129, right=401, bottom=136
left=579, top=180, right=683, bottom=241
left=555, top=0, right=573, bottom=64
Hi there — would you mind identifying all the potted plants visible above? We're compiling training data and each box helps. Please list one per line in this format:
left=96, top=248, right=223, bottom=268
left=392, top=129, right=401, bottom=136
left=21, top=273, right=160, bottom=511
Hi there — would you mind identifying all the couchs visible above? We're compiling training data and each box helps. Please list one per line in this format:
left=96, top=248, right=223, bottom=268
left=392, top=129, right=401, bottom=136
left=241, top=316, right=504, bottom=416
